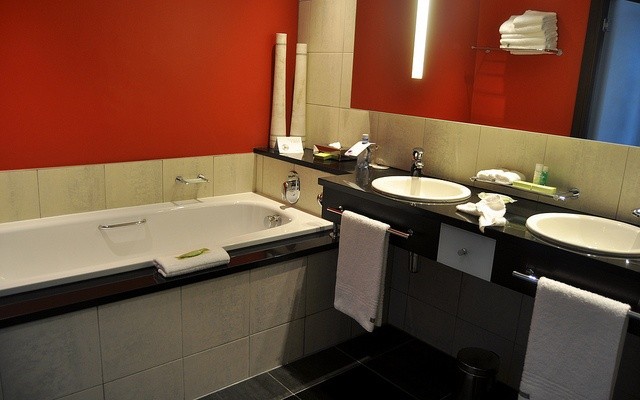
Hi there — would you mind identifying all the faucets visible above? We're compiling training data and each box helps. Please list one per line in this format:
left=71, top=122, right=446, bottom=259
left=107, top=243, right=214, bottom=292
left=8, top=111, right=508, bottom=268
left=631, top=206, right=640, bottom=218
left=281, top=180, right=291, bottom=201
left=411, top=146, right=425, bottom=171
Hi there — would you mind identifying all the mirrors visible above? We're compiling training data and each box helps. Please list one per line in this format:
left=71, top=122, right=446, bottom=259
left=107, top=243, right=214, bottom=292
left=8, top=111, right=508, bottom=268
left=349, top=1, right=592, bottom=139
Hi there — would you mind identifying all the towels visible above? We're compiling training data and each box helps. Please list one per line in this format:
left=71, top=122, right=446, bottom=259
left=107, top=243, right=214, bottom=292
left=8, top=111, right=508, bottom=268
left=455, top=190, right=518, bottom=237
left=333, top=209, right=391, bottom=333
left=498, top=7, right=559, bottom=56
left=475, top=160, right=527, bottom=187
left=153, top=244, right=233, bottom=278
left=517, top=275, right=632, bottom=400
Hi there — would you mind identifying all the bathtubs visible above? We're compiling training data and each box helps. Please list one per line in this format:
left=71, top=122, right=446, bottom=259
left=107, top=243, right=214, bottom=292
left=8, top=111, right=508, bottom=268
left=0, top=191, right=334, bottom=299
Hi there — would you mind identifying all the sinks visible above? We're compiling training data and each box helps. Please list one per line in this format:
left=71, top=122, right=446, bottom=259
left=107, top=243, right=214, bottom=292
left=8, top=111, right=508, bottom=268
left=371, top=175, right=472, bottom=204
left=524, top=212, right=640, bottom=257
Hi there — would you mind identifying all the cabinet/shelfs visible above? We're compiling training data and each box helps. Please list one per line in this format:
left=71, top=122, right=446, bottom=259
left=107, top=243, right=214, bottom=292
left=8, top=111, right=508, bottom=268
left=316, top=177, right=640, bottom=335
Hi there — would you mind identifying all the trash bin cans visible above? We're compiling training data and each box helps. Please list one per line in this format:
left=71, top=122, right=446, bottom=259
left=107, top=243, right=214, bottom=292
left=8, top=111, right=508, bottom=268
left=458, top=349, right=500, bottom=400
